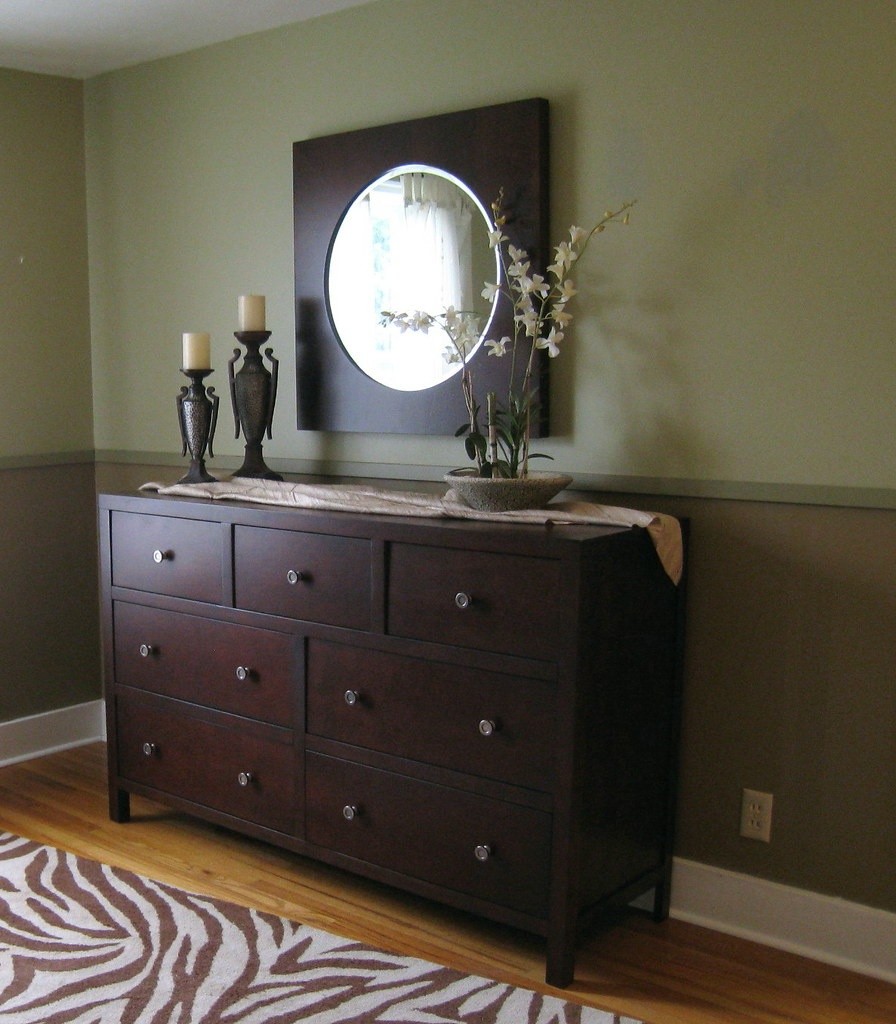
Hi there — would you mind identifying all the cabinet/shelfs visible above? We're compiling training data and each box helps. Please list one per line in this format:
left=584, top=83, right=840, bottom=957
left=97, top=492, right=684, bottom=992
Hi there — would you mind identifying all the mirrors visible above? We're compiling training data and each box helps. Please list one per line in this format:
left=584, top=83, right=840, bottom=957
left=293, top=96, right=549, bottom=438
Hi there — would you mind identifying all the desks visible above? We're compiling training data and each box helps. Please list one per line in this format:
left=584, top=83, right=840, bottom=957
left=0, top=828, right=646, bottom=1024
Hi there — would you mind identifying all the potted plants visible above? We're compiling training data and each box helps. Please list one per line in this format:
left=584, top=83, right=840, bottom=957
left=238, top=296, right=266, bottom=332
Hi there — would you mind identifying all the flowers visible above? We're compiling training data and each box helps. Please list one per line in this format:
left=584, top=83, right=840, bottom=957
left=378, top=182, right=636, bottom=480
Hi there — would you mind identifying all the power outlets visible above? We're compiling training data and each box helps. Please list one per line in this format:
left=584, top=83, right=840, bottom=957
left=741, top=788, right=772, bottom=843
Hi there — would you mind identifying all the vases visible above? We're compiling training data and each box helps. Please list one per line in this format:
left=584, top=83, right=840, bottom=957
left=444, top=473, right=572, bottom=511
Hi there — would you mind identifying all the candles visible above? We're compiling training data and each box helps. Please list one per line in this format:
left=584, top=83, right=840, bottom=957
left=182, top=332, right=211, bottom=369
